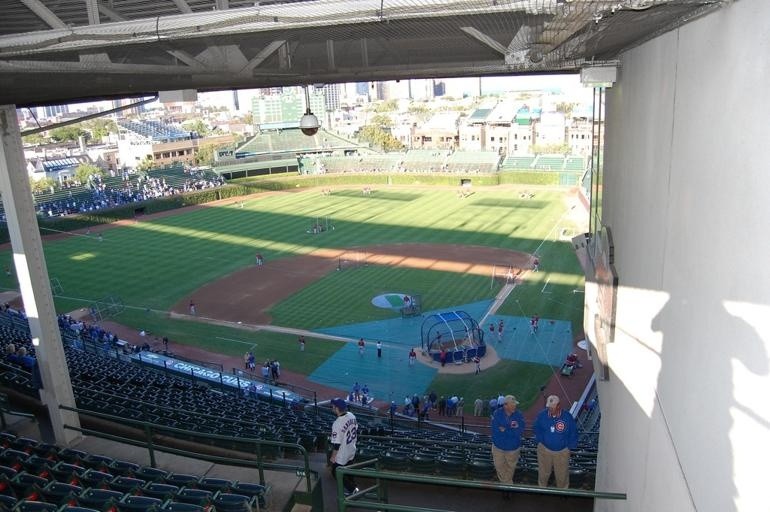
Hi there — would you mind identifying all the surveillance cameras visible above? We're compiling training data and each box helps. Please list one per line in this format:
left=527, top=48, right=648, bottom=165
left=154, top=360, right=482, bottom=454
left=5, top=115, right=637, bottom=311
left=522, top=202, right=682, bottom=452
left=298, top=113, right=321, bottom=136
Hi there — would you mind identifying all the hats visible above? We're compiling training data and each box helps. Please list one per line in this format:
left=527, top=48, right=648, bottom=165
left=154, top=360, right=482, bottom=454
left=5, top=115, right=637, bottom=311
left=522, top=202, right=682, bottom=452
left=330, top=398, right=347, bottom=411
left=546, top=395, right=560, bottom=408
left=505, top=395, right=519, bottom=405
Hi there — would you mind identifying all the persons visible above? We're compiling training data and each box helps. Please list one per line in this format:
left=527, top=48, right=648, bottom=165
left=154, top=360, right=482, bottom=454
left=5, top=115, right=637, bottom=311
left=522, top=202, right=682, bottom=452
left=0, top=302, right=169, bottom=369
left=256, top=252, right=263, bottom=270
left=31, top=159, right=225, bottom=220
left=328, top=397, right=360, bottom=495
left=487, top=314, right=540, bottom=342
left=404, top=295, right=410, bottom=304
left=345, top=380, right=505, bottom=421
left=298, top=334, right=305, bottom=352
left=243, top=349, right=281, bottom=380
left=533, top=394, right=578, bottom=489
left=533, top=257, right=539, bottom=272
left=490, top=394, right=526, bottom=485
left=189, top=300, right=195, bottom=316
left=358, top=330, right=480, bottom=375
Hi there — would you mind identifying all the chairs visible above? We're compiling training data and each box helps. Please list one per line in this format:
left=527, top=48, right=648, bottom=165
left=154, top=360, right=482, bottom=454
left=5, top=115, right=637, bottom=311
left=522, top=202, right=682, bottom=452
left=0, top=160, right=232, bottom=245
left=0, top=300, right=602, bottom=512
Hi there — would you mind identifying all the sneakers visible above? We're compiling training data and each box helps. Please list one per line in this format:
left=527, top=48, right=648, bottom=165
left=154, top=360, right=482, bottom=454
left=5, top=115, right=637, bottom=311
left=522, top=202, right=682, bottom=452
left=344, top=487, right=359, bottom=497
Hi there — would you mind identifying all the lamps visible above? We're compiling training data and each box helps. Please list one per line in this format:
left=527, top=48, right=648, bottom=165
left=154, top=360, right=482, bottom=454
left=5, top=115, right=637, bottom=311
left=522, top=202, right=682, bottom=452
left=579, top=65, right=618, bottom=89
left=157, top=87, right=199, bottom=109
